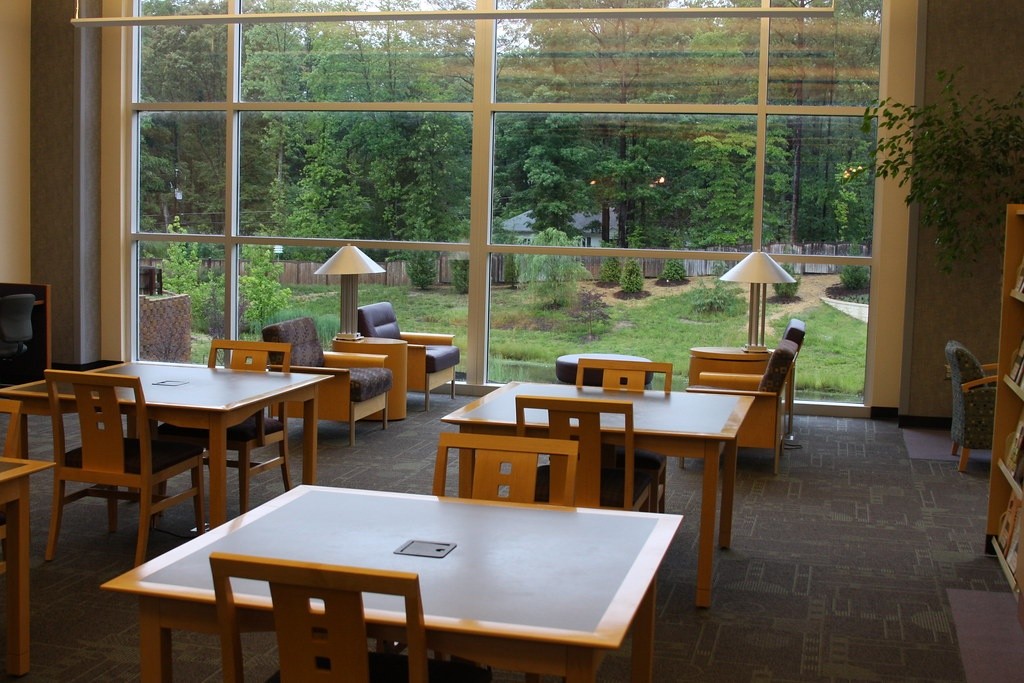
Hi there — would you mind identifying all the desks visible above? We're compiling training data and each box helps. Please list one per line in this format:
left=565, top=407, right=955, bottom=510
left=440, top=381, right=755, bottom=607
left=332, top=336, right=408, bottom=420
left=0, top=457, right=57, bottom=674
left=102, top=485, right=682, bottom=683
left=0, top=360, right=334, bottom=529
left=555, top=353, right=653, bottom=390
left=689, top=346, right=776, bottom=386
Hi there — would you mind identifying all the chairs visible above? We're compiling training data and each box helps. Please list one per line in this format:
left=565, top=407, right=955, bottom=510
left=0, top=399, right=23, bottom=575
left=431, top=433, right=578, bottom=506
left=210, top=553, right=491, bottom=683
left=0, top=292, right=34, bottom=386
left=781, top=318, right=806, bottom=439
left=543, top=358, right=673, bottom=514
left=945, top=339, right=997, bottom=472
left=357, top=302, right=459, bottom=412
left=262, top=317, right=394, bottom=446
left=515, top=396, right=651, bottom=512
left=159, top=340, right=293, bottom=516
left=45, top=369, right=205, bottom=568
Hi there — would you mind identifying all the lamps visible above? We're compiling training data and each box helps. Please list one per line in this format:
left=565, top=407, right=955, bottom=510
left=720, top=249, right=797, bottom=353
left=313, top=244, right=386, bottom=341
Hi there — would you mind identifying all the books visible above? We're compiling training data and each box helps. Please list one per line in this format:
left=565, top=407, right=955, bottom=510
left=997, top=256, right=1024, bottom=584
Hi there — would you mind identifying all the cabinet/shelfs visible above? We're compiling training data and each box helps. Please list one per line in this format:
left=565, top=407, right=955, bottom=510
left=985, top=202, right=1024, bottom=597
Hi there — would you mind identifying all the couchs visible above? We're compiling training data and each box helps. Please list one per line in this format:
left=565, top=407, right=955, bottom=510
left=679, top=340, right=799, bottom=474
